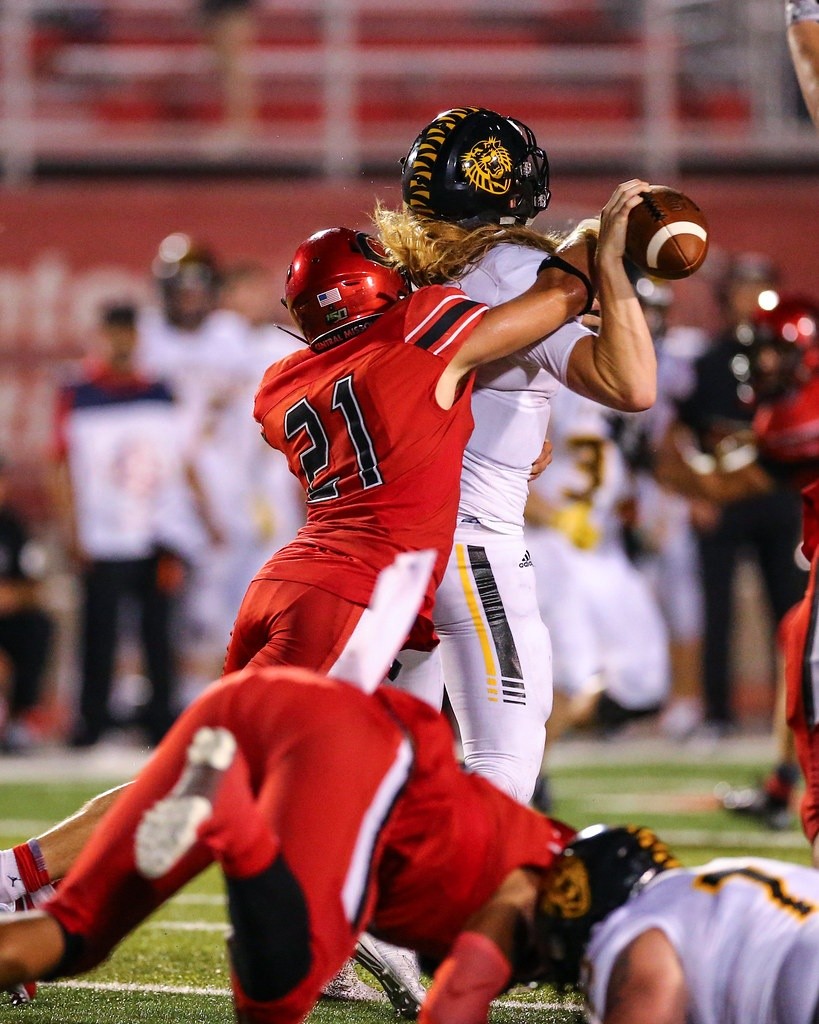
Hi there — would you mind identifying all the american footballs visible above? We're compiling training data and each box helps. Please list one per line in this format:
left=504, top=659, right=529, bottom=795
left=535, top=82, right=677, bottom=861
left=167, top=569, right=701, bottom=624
left=624, top=184, right=709, bottom=281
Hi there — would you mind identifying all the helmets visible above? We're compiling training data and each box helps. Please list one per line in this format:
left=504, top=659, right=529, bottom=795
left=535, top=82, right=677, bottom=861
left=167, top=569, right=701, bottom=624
left=283, top=226, right=412, bottom=350
left=535, top=820, right=677, bottom=995
left=399, top=107, right=550, bottom=228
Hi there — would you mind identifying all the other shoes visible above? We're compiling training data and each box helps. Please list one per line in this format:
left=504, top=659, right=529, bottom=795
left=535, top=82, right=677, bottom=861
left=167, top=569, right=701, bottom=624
left=132, top=726, right=250, bottom=881
left=721, top=790, right=788, bottom=833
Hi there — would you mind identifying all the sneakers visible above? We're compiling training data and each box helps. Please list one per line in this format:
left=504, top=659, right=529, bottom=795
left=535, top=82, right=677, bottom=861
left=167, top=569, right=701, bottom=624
left=354, top=930, right=428, bottom=1020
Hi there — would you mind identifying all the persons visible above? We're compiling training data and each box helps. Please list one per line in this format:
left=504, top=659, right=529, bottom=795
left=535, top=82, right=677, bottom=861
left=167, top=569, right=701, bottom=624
left=538, top=819, right=819, bottom=1024
left=0, top=229, right=318, bottom=757
left=518, top=249, right=819, bottom=859
left=0, top=669, right=581, bottom=1024
left=316, top=104, right=657, bottom=1023
left=1, top=213, right=604, bottom=999
